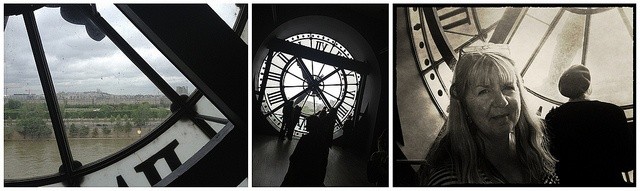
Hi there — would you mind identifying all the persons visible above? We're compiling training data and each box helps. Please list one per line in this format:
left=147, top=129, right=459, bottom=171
left=541, top=64, right=632, bottom=183
left=282, top=100, right=338, bottom=187
left=343, top=115, right=352, bottom=139
left=414, top=44, right=558, bottom=186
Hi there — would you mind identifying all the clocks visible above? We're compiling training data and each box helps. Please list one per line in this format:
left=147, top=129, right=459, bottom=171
left=405, top=6, right=633, bottom=185
left=256, top=34, right=362, bottom=138
left=4, top=3, right=249, bottom=188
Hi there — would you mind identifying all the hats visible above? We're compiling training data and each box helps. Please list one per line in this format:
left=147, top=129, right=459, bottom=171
left=558, top=64, right=590, bottom=98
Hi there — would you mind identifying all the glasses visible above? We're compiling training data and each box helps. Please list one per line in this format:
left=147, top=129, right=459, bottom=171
left=459, top=44, right=511, bottom=60
